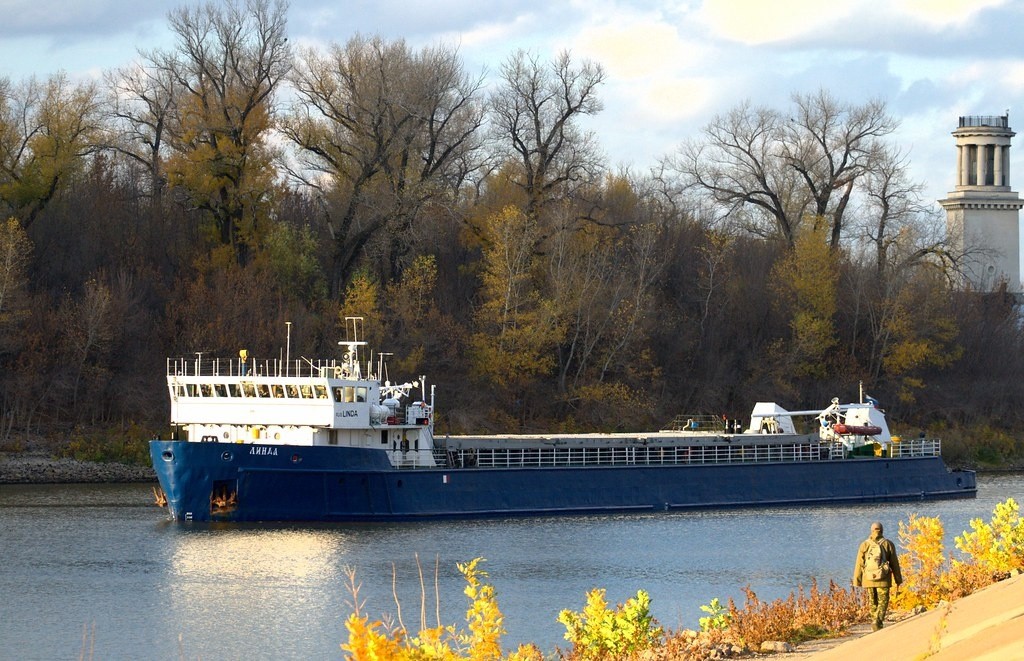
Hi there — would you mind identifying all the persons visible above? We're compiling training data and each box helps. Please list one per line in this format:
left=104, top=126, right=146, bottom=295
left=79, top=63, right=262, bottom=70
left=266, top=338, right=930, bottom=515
left=852, top=523, right=902, bottom=633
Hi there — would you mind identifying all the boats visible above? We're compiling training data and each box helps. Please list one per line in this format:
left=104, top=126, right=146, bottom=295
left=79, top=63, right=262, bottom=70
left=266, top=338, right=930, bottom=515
left=150, top=317, right=978, bottom=524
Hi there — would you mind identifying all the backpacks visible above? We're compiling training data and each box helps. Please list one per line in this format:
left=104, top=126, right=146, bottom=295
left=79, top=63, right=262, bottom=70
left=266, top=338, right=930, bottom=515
left=864, top=538, right=890, bottom=582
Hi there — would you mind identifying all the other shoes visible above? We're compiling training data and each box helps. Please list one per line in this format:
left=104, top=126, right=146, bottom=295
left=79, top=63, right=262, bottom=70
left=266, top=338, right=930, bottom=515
left=871, top=621, right=883, bottom=632
